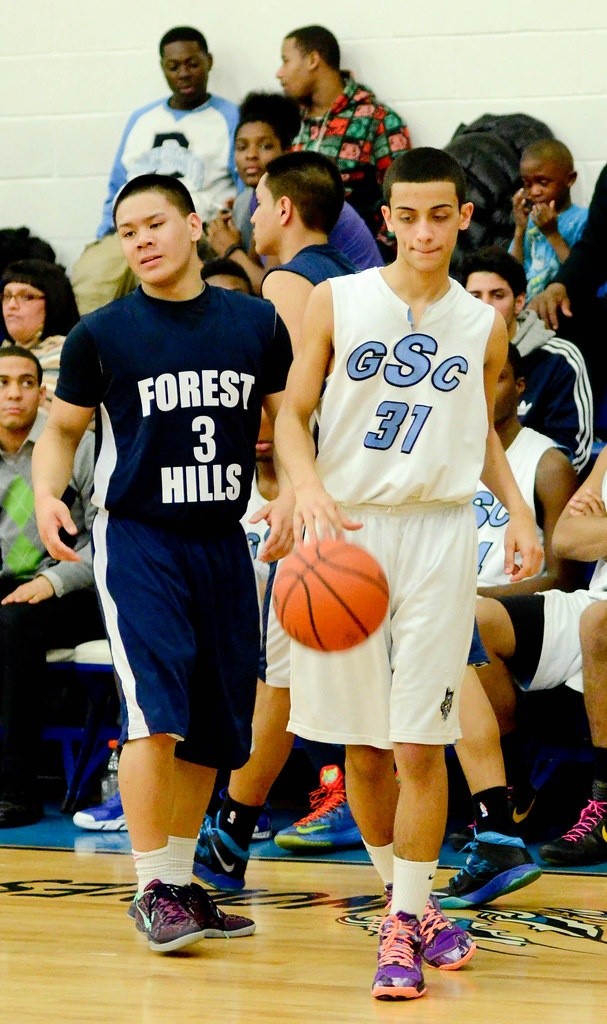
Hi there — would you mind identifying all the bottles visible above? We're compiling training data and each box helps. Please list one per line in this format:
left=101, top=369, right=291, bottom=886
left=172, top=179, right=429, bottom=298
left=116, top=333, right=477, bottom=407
left=99, top=739, right=121, bottom=804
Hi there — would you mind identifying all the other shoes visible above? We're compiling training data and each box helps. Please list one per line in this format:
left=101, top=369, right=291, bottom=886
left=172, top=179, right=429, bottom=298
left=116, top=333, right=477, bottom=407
left=250, top=813, right=271, bottom=839
left=450, top=788, right=542, bottom=850
left=72, top=792, right=127, bottom=832
left=0, top=790, right=45, bottom=828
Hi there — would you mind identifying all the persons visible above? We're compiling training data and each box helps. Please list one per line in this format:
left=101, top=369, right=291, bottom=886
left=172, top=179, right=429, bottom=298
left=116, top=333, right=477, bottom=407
left=96, top=24, right=245, bottom=232
left=31, top=173, right=296, bottom=952
left=509, top=141, right=589, bottom=302
left=451, top=446, right=607, bottom=866
left=276, top=25, right=413, bottom=248
left=274, top=146, right=545, bottom=996
left=192, top=150, right=544, bottom=908
left=529, top=167, right=607, bottom=443
left=207, top=92, right=384, bottom=273
left=0, top=226, right=593, bottom=851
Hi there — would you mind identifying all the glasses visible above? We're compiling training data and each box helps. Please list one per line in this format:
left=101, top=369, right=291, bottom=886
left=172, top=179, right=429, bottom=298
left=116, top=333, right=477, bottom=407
left=0, top=287, right=45, bottom=305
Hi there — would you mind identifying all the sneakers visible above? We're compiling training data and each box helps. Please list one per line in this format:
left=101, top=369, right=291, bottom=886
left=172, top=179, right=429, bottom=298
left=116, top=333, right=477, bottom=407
left=539, top=780, right=607, bottom=866
left=369, top=911, right=427, bottom=1001
left=192, top=811, right=251, bottom=894
left=382, top=881, right=477, bottom=970
left=128, top=881, right=205, bottom=953
left=428, top=825, right=542, bottom=909
left=274, top=765, right=363, bottom=851
left=166, top=882, right=256, bottom=938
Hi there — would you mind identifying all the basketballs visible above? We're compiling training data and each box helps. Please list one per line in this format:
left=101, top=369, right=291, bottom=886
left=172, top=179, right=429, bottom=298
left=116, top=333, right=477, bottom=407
left=274, top=539, right=389, bottom=652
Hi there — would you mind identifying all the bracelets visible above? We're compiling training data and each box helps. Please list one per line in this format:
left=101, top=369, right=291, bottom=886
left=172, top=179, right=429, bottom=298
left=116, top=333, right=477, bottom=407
left=225, top=243, right=245, bottom=258
